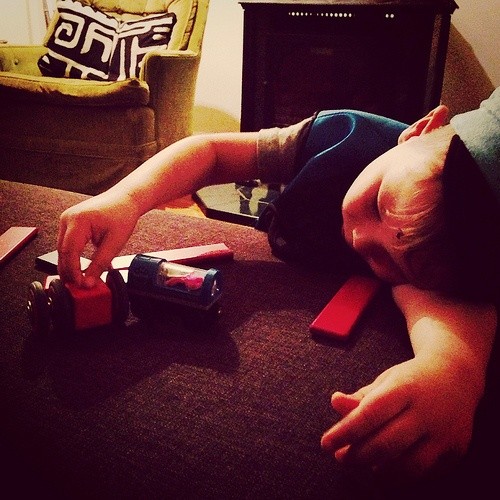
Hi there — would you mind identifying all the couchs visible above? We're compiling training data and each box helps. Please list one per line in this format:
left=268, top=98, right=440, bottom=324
left=1, top=0, right=211, bottom=190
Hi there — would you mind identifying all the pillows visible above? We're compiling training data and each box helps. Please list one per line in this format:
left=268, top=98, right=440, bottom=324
left=38, top=0, right=177, bottom=81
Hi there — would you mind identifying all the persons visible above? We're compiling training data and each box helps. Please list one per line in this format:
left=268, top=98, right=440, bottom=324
left=59, top=88, right=500, bottom=483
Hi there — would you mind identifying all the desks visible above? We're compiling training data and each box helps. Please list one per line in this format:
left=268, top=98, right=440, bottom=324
left=0, top=177, right=500, bottom=500
